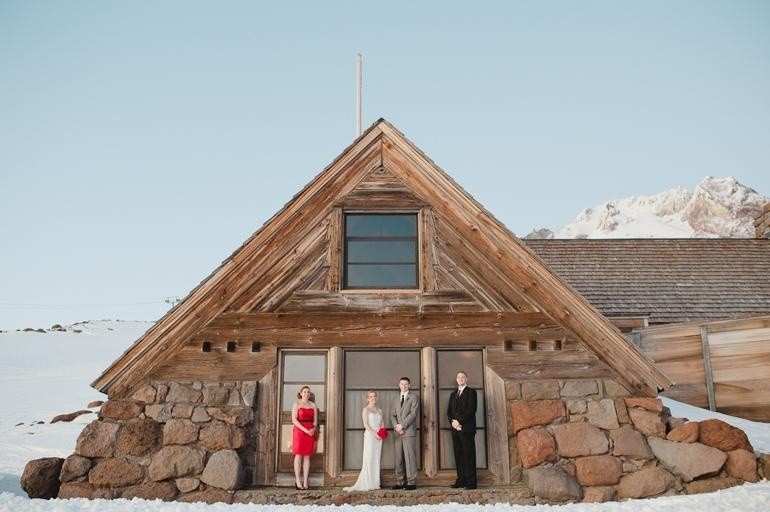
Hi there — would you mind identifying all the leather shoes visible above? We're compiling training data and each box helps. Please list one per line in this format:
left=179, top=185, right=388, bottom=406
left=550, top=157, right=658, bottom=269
left=450, top=481, right=477, bottom=489
left=295, top=482, right=309, bottom=490
left=393, top=484, right=417, bottom=490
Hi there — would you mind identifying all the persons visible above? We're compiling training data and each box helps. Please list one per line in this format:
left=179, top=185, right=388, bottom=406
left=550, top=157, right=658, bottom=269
left=390, top=377, right=419, bottom=490
left=446, top=370, right=478, bottom=490
left=292, top=386, right=318, bottom=490
left=343, top=389, right=386, bottom=492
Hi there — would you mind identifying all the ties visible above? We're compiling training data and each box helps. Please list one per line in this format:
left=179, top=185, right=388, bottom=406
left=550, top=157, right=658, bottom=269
left=400, top=395, right=404, bottom=408
left=456, top=390, right=461, bottom=400
